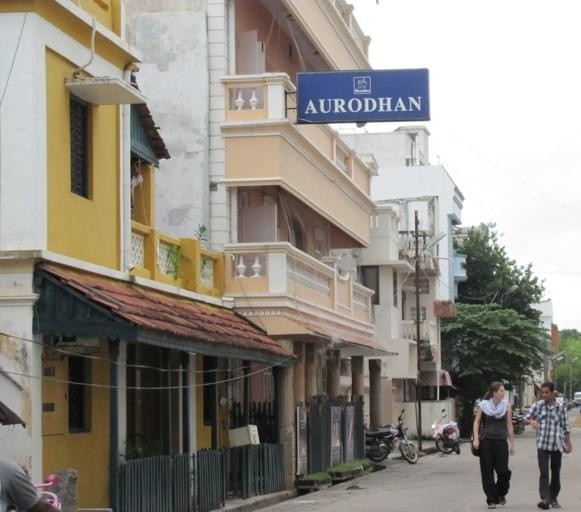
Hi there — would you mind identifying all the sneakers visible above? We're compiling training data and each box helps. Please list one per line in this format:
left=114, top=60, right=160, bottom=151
left=537, top=497, right=550, bottom=510
left=498, top=496, right=507, bottom=505
left=486, top=499, right=497, bottom=510
left=550, top=496, right=561, bottom=508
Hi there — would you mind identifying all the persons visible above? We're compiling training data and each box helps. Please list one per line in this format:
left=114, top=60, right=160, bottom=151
left=473, top=381, right=516, bottom=509
left=0, top=457, right=60, bottom=512
left=521, top=383, right=571, bottom=509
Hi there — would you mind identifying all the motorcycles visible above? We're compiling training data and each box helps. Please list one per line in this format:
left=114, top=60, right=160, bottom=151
left=366, top=408, right=418, bottom=465
left=436, top=409, right=461, bottom=454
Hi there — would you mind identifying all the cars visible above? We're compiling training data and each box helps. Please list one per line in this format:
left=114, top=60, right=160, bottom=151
left=574, top=391, right=581, bottom=405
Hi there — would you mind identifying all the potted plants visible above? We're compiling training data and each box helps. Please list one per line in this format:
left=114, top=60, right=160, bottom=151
left=293, top=460, right=363, bottom=492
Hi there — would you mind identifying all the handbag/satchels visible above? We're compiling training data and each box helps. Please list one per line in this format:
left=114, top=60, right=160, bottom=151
left=471, top=438, right=483, bottom=457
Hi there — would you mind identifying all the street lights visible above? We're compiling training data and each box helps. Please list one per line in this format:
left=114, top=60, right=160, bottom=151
left=547, top=351, right=565, bottom=383
left=415, top=210, right=448, bottom=451
